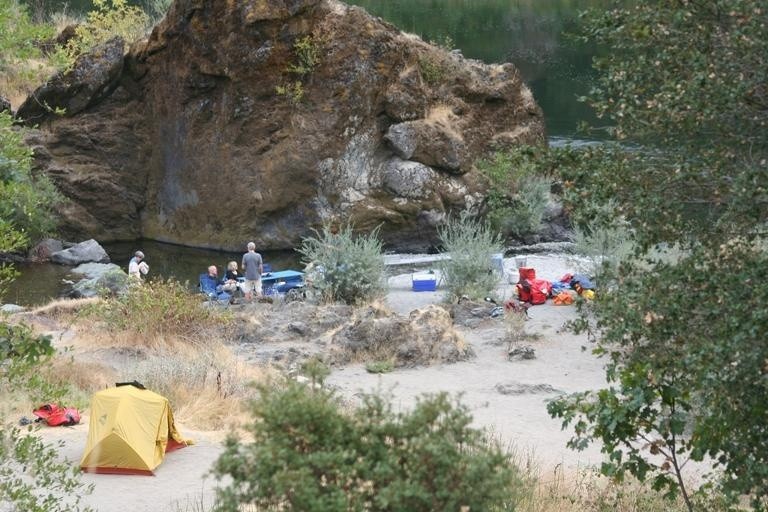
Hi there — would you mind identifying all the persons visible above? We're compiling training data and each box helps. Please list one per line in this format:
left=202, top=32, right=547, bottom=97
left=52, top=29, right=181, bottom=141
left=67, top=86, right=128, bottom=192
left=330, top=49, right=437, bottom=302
left=128, top=250, right=146, bottom=281
left=225, top=261, right=243, bottom=291
left=240, top=241, right=264, bottom=300
left=207, top=262, right=236, bottom=304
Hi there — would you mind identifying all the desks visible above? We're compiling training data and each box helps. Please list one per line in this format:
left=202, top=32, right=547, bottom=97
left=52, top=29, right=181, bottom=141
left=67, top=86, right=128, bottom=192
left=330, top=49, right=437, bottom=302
left=236, top=270, right=306, bottom=297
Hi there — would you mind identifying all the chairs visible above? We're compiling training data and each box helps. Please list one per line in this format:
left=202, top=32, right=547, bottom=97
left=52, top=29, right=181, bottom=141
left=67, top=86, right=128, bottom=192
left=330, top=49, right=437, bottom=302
left=262, top=263, right=279, bottom=288
left=200, top=273, right=234, bottom=305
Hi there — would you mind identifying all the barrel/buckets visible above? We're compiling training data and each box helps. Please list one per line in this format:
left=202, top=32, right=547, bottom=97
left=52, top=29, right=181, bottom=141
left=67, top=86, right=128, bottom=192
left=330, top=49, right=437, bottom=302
left=515, top=256, right=526, bottom=268
left=509, top=273, right=520, bottom=284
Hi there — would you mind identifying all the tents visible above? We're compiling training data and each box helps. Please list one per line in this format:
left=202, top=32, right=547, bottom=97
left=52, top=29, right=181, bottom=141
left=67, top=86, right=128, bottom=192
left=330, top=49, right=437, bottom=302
left=78, top=381, right=194, bottom=476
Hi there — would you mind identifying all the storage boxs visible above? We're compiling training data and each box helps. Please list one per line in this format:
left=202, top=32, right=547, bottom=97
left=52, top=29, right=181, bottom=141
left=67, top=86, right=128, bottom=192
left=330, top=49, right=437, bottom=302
left=412, top=274, right=436, bottom=292
